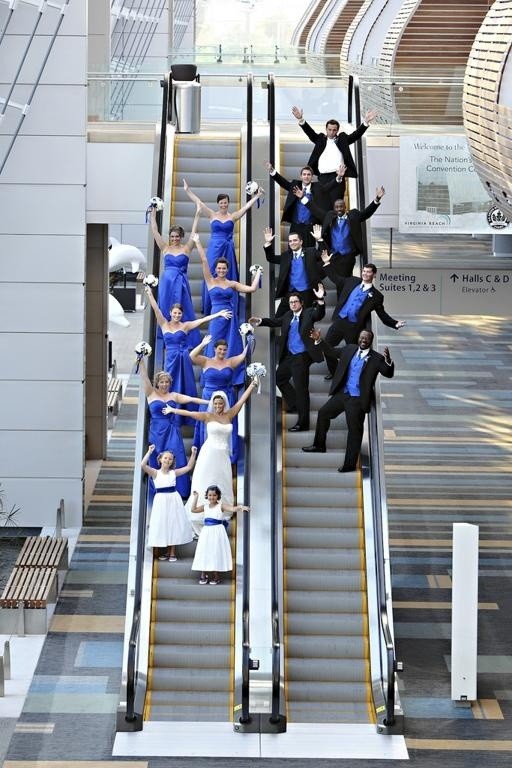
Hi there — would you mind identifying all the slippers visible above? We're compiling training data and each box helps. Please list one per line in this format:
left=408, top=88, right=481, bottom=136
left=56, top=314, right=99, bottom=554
left=168, top=555, right=177, bottom=561
left=158, top=554, right=169, bottom=561
left=199, top=574, right=209, bottom=584
left=209, top=578, right=221, bottom=585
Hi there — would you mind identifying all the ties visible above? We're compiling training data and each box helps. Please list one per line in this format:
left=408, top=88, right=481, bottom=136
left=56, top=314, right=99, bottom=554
left=357, top=351, right=362, bottom=359
left=293, top=315, right=297, bottom=321
left=360, top=284, right=364, bottom=291
left=338, top=218, right=343, bottom=226
left=303, top=188, right=306, bottom=194
left=295, top=253, right=297, bottom=260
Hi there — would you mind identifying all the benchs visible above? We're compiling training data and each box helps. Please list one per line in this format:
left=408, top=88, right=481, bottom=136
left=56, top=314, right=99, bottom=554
left=16, top=535, right=69, bottom=601
left=1, top=566, right=59, bottom=638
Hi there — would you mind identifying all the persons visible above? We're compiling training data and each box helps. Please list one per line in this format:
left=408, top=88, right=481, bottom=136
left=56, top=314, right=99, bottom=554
left=137, top=180, right=265, bottom=585
left=248, top=105, right=406, bottom=472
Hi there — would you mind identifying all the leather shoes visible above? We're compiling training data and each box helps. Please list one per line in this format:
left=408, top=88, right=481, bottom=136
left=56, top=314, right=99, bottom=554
left=302, top=445, right=326, bottom=452
left=288, top=424, right=310, bottom=432
left=287, top=406, right=297, bottom=414
left=338, top=464, right=356, bottom=472
left=325, top=373, right=332, bottom=380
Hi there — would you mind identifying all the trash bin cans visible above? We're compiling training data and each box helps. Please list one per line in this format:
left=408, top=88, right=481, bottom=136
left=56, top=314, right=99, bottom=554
left=172, top=81, right=201, bottom=134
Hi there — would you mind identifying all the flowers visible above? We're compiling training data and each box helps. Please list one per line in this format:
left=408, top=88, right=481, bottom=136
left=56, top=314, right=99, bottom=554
left=144, top=197, right=165, bottom=222
left=249, top=264, right=264, bottom=282
left=246, top=361, right=267, bottom=388
left=140, top=273, right=160, bottom=297
left=245, top=180, right=265, bottom=209
left=238, top=322, right=255, bottom=341
left=132, top=340, right=152, bottom=376
